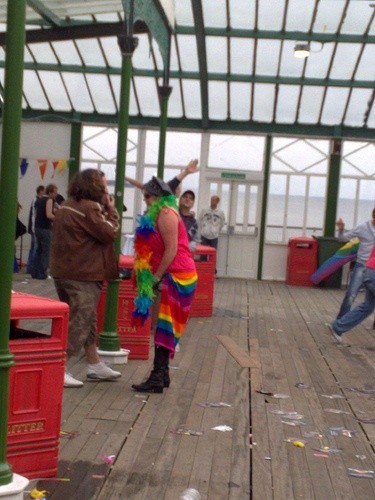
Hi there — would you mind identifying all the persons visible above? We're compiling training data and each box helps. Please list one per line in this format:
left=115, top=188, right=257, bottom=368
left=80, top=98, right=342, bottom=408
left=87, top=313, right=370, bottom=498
left=14, top=202, right=22, bottom=273
left=26, top=184, right=65, bottom=279
left=328, top=209, right=375, bottom=344
left=200, top=195, right=226, bottom=279
left=169, top=159, right=201, bottom=244
left=132, top=176, right=198, bottom=392
left=48, top=168, right=123, bottom=389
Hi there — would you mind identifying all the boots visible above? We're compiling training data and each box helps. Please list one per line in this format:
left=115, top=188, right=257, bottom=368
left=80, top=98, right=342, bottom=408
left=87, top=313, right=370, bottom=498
left=132, top=368, right=170, bottom=392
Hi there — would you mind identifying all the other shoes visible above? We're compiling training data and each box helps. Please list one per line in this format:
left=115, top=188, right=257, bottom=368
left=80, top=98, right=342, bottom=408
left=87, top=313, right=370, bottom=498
left=327, top=324, right=341, bottom=341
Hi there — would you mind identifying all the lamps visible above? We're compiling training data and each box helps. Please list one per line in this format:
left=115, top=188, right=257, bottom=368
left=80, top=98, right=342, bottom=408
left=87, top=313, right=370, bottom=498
left=294, top=44, right=310, bottom=58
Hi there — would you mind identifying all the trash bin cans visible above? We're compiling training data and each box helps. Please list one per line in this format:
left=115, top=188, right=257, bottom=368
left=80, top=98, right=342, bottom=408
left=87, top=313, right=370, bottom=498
left=96, top=255, right=152, bottom=362
left=8, top=291, right=69, bottom=479
left=175, top=244, right=216, bottom=317
left=286, top=237, right=317, bottom=289
left=312, top=235, right=350, bottom=288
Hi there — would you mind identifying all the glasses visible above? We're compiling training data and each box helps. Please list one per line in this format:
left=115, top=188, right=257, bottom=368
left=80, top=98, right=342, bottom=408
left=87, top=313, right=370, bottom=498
left=144, top=194, right=151, bottom=199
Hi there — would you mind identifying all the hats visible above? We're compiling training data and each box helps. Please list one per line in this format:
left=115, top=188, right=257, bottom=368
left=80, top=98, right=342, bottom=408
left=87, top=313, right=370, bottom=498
left=144, top=176, right=172, bottom=196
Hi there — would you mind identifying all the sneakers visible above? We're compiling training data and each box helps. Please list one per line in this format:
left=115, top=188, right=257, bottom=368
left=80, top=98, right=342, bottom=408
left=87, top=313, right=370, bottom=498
left=87, top=366, right=121, bottom=380
left=63, top=374, right=83, bottom=388
